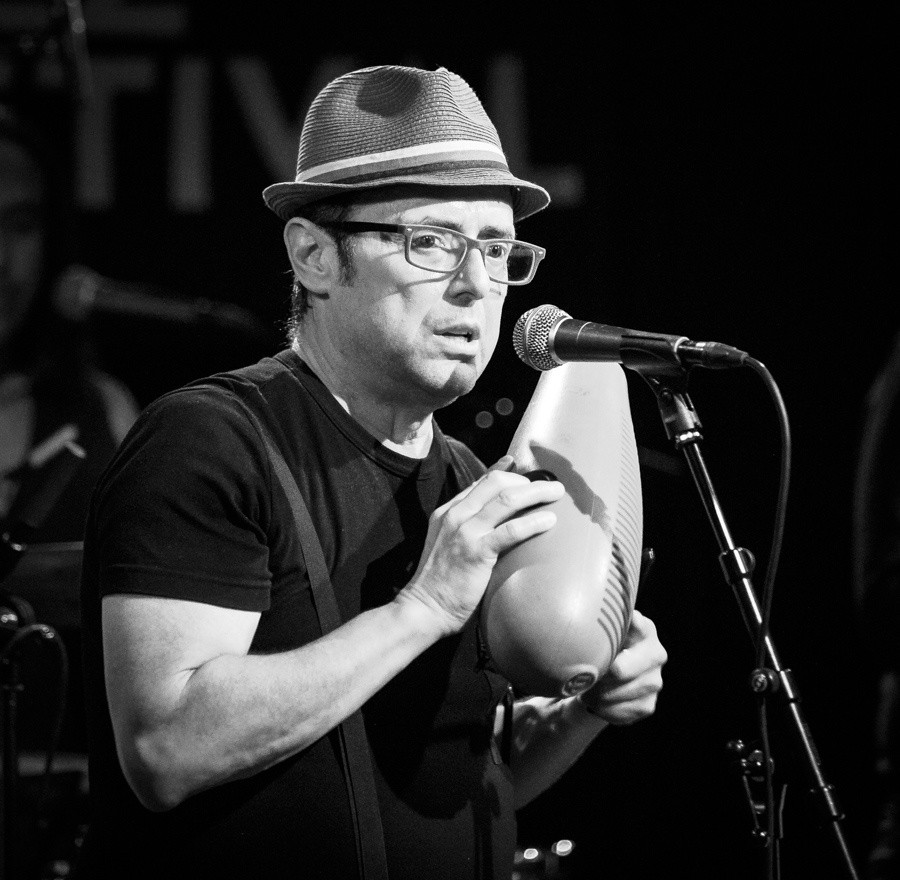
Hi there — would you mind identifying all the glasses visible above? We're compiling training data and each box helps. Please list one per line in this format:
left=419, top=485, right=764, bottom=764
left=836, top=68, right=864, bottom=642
left=318, top=220, right=547, bottom=286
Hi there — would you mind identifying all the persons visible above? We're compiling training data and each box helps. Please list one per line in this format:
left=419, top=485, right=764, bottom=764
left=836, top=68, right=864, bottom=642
left=78, top=68, right=667, bottom=880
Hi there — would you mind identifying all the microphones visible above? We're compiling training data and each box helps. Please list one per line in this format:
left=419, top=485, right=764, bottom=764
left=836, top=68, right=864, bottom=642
left=513, top=305, right=750, bottom=368
left=51, top=264, right=254, bottom=330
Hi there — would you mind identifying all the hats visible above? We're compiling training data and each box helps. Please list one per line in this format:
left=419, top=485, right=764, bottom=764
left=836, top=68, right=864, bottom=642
left=262, top=65, right=550, bottom=222
